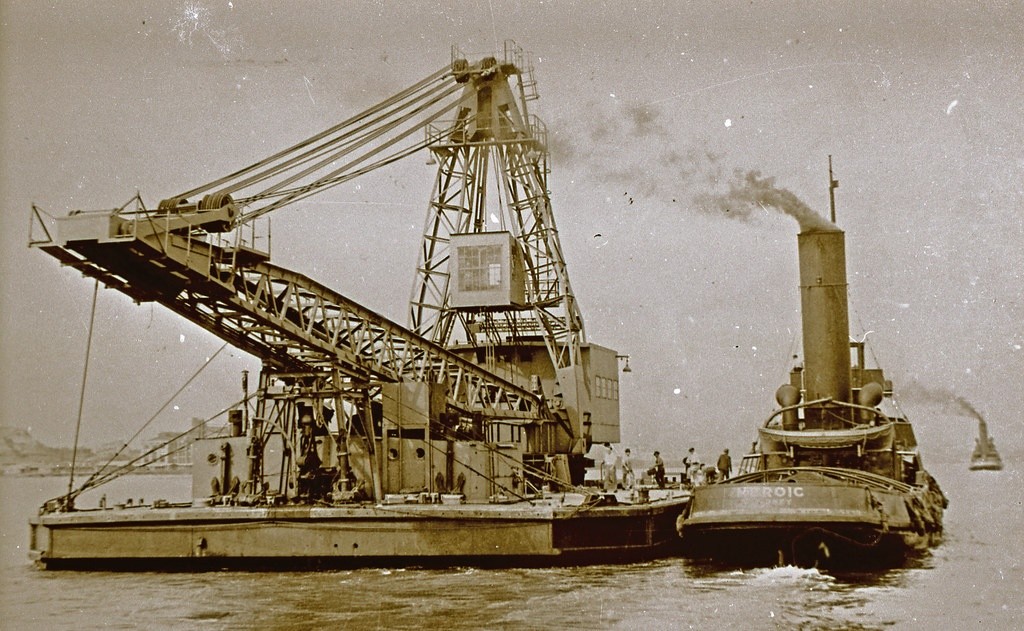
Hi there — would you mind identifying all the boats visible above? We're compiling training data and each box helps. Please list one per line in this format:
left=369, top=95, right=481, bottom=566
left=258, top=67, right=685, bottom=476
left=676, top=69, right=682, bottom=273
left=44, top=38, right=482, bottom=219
left=969, top=419, right=1003, bottom=470
left=679, top=154, right=945, bottom=562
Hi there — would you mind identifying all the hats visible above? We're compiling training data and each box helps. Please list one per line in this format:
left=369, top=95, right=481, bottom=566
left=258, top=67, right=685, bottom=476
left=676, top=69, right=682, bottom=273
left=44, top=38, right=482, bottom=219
left=688, top=447, right=694, bottom=452
left=625, top=448, right=630, bottom=452
left=653, top=451, right=660, bottom=455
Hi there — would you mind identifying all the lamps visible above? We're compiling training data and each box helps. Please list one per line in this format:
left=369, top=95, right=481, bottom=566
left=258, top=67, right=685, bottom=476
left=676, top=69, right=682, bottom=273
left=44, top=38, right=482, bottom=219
left=615, top=355, right=631, bottom=372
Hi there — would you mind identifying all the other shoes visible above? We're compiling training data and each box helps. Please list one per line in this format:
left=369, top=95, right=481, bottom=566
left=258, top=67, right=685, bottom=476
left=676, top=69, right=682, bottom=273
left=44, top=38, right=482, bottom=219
left=614, top=490, right=617, bottom=493
left=601, top=489, right=607, bottom=492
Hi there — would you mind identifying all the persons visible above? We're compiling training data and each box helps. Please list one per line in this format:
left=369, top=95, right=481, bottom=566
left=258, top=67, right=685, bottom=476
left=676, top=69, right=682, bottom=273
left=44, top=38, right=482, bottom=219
left=621, top=449, right=635, bottom=490
left=601, top=447, right=618, bottom=493
left=652, top=449, right=666, bottom=490
left=684, top=447, right=732, bottom=486
